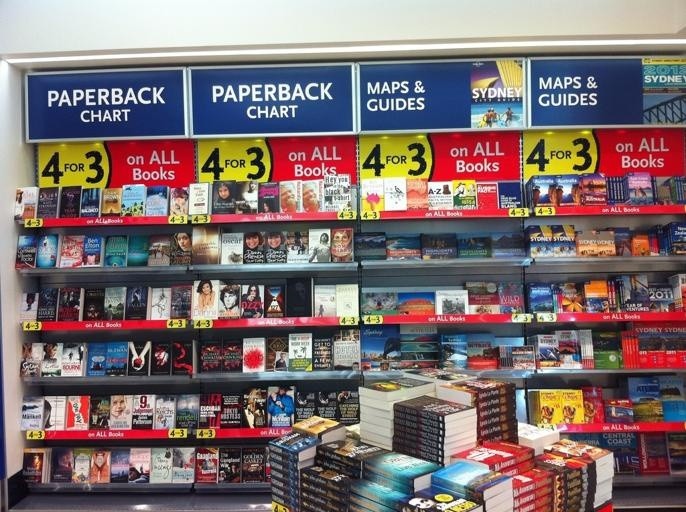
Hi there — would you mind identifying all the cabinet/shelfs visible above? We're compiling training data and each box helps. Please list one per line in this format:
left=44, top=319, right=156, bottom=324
left=355, top=56, right=535, bottom=424
left=189, top=61, right=363, bottom=494
left=523, top=55, right=686, bottom=488
left=16, top=65, right=199, bottom=492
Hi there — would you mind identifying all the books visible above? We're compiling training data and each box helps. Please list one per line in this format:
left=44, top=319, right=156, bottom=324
left=13, top=172, right=686, bottom=512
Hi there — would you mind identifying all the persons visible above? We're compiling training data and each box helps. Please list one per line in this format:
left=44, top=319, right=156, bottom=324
left=483, top=107, right=513, bottom=127
